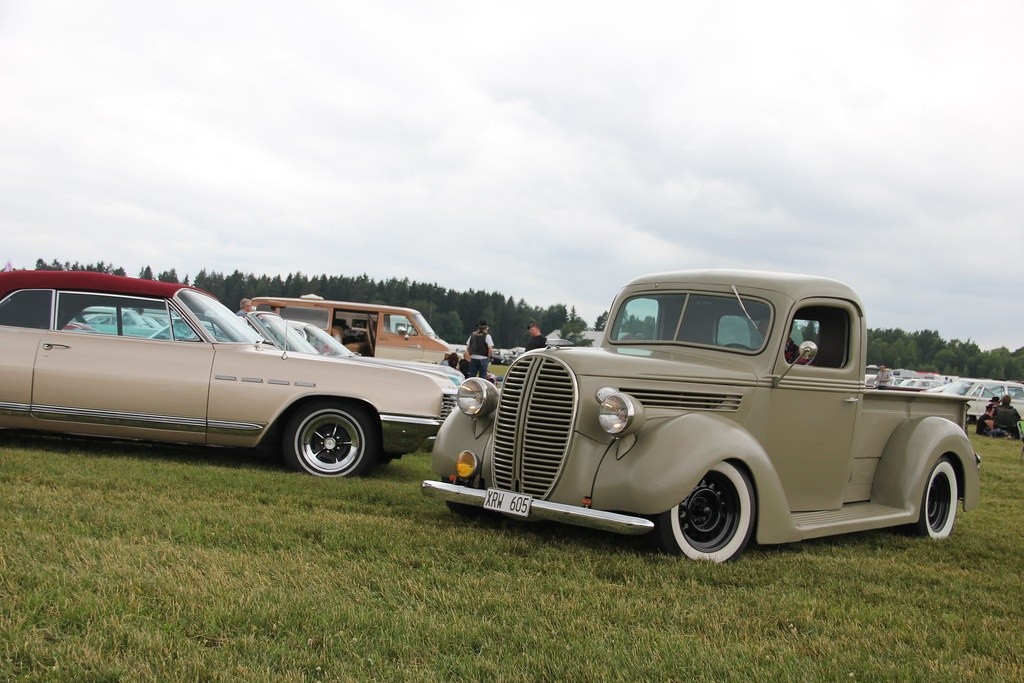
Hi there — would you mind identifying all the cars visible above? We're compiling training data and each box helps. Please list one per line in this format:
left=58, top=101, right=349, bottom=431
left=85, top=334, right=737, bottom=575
left=68, top=302, right=359, bottom=360
left=862, top=363, right=1024, bottom=418
left=0, top=268, right=460, bottom=477
left=486, top=346, right=529, bottom=366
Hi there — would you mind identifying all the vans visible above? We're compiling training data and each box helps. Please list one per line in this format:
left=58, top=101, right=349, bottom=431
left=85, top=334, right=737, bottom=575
left=249, top=293, right=454, bottom=371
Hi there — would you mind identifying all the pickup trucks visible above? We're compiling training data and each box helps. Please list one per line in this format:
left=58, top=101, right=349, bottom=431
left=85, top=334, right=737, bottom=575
left=413, top=265, right=987, bottom=566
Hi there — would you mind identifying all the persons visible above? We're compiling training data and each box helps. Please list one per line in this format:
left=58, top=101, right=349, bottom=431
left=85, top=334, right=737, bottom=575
left=975, top=403, right=1009, bottom=438
left=944, top=377, right=950, bottom=384
left=873, top=365, right=891, bottom=386
left=442, top=353, right=458, bottom=369
left=459, top=320, right=494, bottom=379
left=754, top=312, right=815, bottom=366
left=989, top=396, right=1002, bottom=408
left=236, top=297, right=253, bottom=316
left=122, top=308, right=145, bottom=327
left=524, top=323, right=547, bottom=351
left=994, top=395, right=1023, bottom=440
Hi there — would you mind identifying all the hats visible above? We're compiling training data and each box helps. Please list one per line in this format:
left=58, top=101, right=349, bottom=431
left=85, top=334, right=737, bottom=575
left=479, top=321, right=487, bottom=327
left=989, top=397, right=1001, bottom=402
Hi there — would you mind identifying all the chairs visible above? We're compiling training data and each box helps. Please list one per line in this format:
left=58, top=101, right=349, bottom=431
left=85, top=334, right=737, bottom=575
left=995, top=406, right=1019, bottom=440
left=331, top=326, right=368, bottom=354
